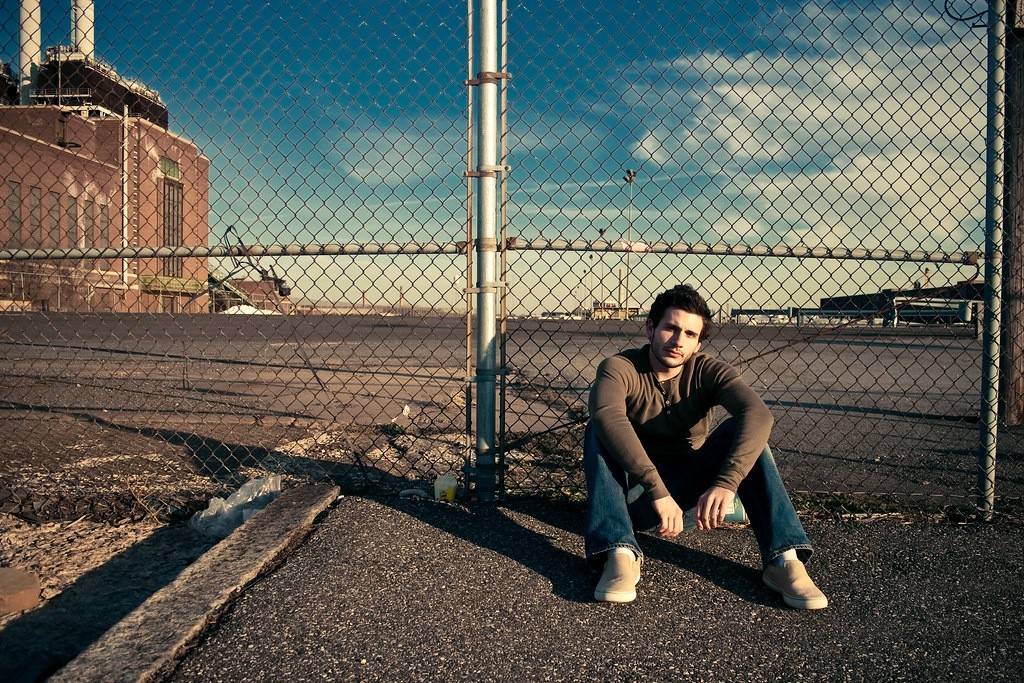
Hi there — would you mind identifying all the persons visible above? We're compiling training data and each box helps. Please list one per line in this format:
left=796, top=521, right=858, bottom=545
left=583, top=285, right=828, bottom=609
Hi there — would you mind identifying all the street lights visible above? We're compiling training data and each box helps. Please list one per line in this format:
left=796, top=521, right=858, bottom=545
left=619, top=167, right=637, bottom=322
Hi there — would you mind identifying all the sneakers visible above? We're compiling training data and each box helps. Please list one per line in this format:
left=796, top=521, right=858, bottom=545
left=594, top=553, right=641, bottom=603
left=762, top=561, right=829, bottom=609
